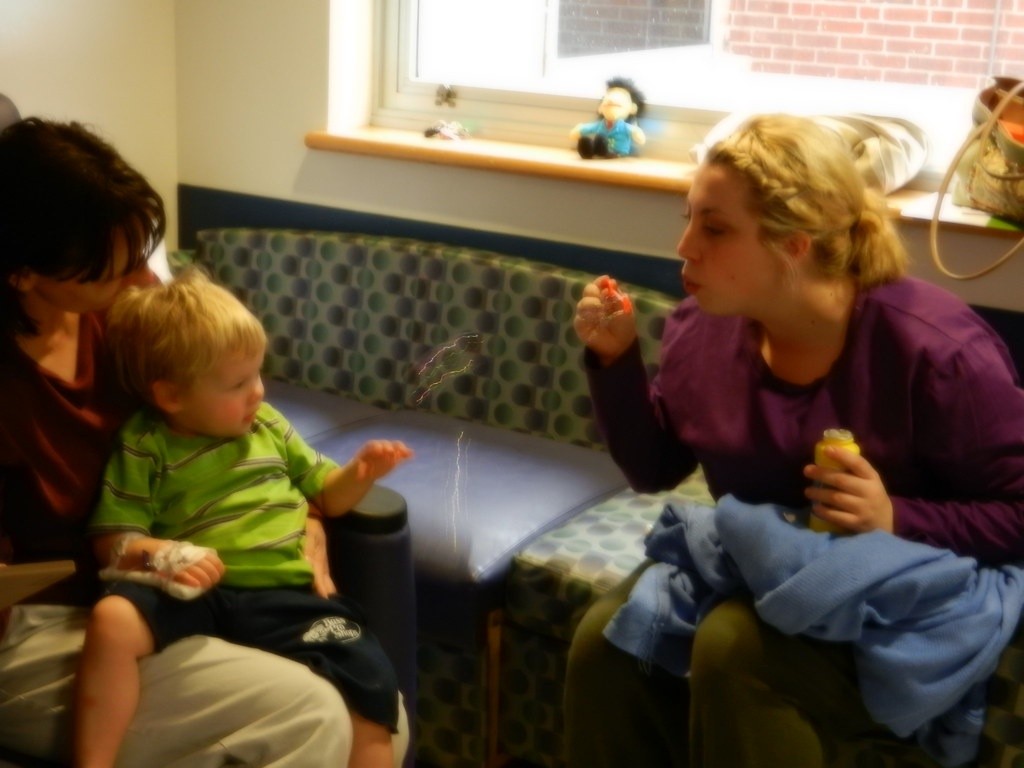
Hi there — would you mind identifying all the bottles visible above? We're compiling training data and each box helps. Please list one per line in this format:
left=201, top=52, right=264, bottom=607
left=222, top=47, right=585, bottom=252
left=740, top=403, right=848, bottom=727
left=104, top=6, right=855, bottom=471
left=812, top=428, right=859, bottom=533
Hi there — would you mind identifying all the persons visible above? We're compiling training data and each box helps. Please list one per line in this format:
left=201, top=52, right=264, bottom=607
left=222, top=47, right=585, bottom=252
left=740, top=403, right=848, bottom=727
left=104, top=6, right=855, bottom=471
left=563, top=111, right=1024, bottom=768
left=573, top=76, right=647, bottom=159
left=0, top=116, right=413, bottom=768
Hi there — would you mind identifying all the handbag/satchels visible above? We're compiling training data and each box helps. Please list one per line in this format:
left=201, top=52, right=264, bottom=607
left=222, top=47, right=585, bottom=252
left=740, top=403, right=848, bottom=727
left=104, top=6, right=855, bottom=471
left=814, top=104, right=932, bottom=195
left=929, top=74, right=1024, bottom=280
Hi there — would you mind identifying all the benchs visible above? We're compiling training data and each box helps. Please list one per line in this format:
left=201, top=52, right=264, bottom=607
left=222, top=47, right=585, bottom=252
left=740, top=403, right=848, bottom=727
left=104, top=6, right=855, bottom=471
left=191, top=224, right=1024, bottom=768
